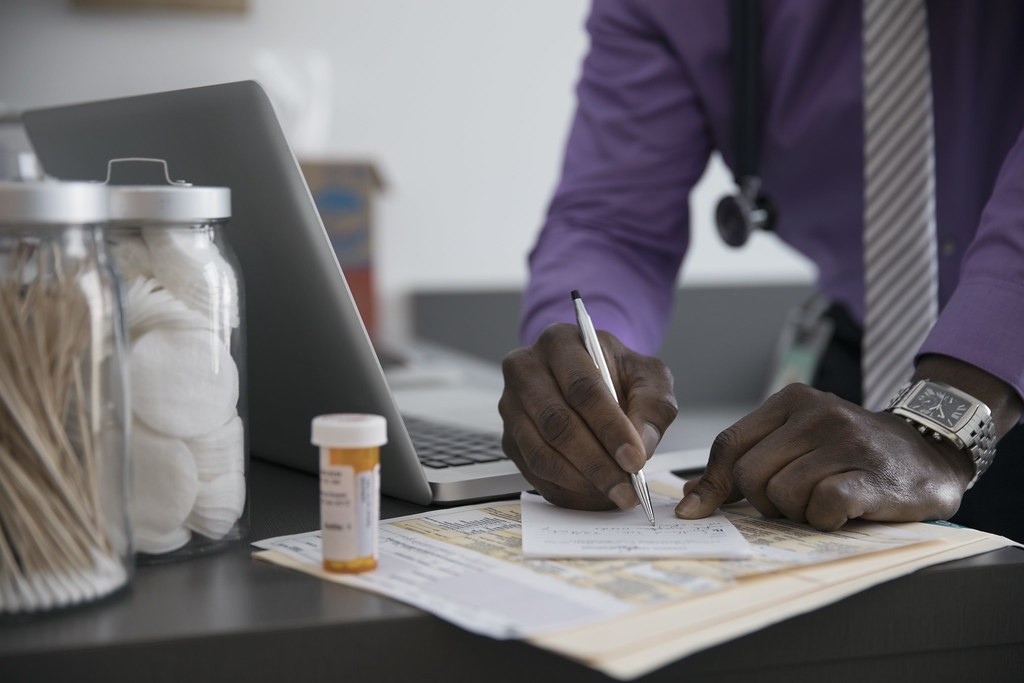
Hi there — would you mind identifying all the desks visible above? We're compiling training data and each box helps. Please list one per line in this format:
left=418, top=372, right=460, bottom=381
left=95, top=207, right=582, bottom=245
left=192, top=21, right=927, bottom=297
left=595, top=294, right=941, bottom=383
left=0, top=460, right=1024, bottom=683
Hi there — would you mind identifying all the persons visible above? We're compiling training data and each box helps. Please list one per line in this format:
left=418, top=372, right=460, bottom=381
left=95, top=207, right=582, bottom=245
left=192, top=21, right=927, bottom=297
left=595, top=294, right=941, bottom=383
left=497, top=1, right=1024, bottom=531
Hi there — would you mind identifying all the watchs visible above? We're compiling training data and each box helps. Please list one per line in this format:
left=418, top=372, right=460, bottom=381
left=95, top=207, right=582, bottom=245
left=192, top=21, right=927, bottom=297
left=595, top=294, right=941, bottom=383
left=884, top=378, right=999, bottom=491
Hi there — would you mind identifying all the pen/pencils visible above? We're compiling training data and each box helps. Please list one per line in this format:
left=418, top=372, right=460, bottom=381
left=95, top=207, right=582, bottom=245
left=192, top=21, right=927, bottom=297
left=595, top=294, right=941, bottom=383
left=570, top=286, right=659, bottom=529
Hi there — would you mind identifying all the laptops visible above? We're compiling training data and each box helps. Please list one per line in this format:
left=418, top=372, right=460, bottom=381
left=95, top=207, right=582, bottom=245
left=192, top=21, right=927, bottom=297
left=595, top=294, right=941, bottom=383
left=0, top=79, right=720, bottom=503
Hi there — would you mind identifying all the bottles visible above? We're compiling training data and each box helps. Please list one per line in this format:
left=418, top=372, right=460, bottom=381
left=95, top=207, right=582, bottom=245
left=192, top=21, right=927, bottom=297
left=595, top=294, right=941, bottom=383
left=0, top=180, right=132, bottom=619
left=108, top=182, right=249, bottom=565
